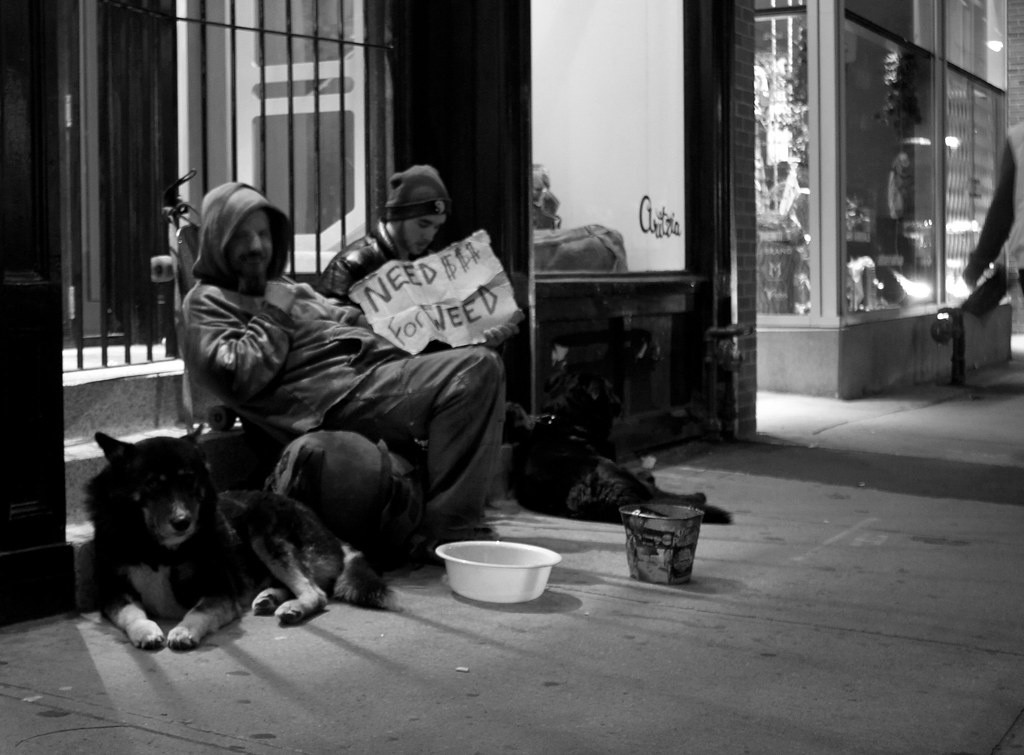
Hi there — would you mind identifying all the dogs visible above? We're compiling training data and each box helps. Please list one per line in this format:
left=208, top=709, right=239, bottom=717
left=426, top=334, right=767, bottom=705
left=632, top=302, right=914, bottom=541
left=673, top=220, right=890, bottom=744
left=84, top=424, right=395, bottom=650
left=511, top=366, right=735, bottom=527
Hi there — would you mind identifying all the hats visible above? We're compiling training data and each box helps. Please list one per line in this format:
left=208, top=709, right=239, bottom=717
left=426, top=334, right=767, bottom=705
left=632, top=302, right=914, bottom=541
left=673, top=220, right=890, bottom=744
left=382, top=164, right=453, bottom=222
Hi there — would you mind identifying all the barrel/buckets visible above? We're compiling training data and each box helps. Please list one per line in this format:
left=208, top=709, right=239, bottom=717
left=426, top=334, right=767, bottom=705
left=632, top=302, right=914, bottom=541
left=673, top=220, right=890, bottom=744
left=620, top=502, right=705, bottom=585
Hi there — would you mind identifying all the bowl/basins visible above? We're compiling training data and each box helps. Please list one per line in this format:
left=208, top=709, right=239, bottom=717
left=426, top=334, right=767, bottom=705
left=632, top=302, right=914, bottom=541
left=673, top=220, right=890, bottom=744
left=433, top=540, right=562, bottom=604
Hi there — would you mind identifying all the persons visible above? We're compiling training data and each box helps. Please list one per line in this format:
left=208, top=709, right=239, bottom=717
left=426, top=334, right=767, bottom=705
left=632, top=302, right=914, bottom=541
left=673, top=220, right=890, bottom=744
left=963, top=120, right=1023, bottom=294
left=314, top=164, right=455, bottom=309
left=174, top=181, right=521, bottom=542
